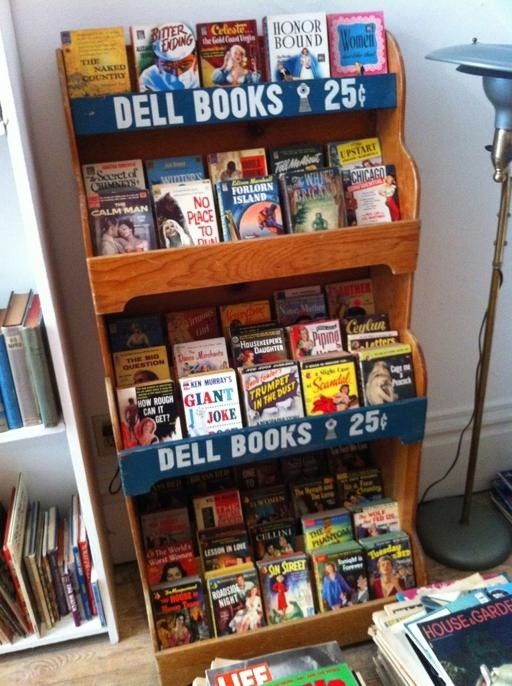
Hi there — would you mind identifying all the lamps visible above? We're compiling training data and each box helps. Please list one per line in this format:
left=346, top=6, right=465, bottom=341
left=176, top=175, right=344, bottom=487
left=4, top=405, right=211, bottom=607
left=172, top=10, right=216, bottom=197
left=417, top=37, right=512, bottom=572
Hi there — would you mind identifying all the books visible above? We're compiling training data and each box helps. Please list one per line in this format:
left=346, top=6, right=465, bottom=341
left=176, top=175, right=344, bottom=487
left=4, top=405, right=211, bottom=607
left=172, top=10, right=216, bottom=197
left=326, top=11, right=388, bottom=76
left=134, top=442, right=418, bottom=650
left=366, top=568, right=512, bottom=684
left=0, top=287, right=61, bottom=430
left=190, top=639, right=366, bottom=685
left=59, top=25, right=133, bottom=100
left=208, top=136, right=403, bottom=242
left=112, top=278, right=416, bottom=449
left=129, top=23, right=201, bottom=93
left=83, top=155, right=219, bottom=256
left=196, top=20, right=264, bottom=88
left=0, top=473, right=107, bottom=644
left=264, top=17, right=332, bottom=83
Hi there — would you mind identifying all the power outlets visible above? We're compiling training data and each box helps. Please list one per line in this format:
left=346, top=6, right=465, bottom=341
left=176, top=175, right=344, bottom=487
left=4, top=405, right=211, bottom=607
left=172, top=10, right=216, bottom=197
left=90, top=413, right=118, bottom=459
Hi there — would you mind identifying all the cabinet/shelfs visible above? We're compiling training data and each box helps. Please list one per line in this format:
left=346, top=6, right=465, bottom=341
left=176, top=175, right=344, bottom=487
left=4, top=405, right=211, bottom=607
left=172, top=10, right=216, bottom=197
left=0, top=0, right=121, bottom=656
left=55, top=30, right=431, bottom=686
